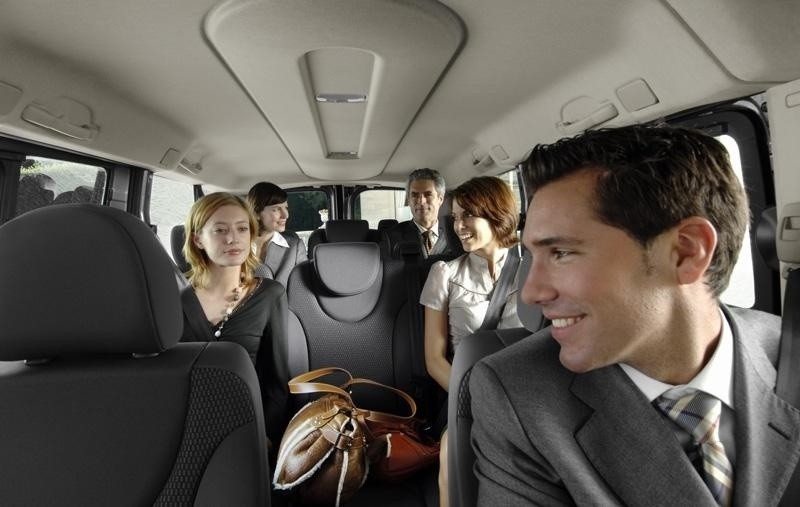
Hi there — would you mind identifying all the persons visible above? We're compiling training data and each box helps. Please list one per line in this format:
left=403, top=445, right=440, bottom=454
left=464, top=121, right=800, bottom=507
left=418, top=173, right=523, bottom=507
left=380, top=165, right=466, bottom=265
left=243, top=179, right=308, bottom=293
left=171, top=191, right=293, bottom=482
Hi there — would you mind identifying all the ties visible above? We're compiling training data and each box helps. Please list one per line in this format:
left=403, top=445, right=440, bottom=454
left=655, top=388, right=735, bottom=506
left=422, top=229, right=436, bottom=256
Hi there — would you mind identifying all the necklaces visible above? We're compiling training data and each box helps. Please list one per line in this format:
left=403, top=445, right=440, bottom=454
left=212, top=280, right=247, bottom=341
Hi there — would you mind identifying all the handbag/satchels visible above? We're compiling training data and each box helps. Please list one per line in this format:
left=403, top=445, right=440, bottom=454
left=271, top=364, right=417, bottom=502
left=366, top=409, right=441, bottom=478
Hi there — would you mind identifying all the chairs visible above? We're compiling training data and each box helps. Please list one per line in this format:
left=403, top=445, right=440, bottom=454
left=4, top=204, right=273, bottom=505
left=445, top=243, right=545, bottom=505
left=382, top=215, right=453, bottom=258
left=285, top=243, right=407, bottom=406
left=406, top=243, right=526, bottom=445
left=171, top=222, right=295, bottom=382
left=308, top=221, right=383, bottom=258
